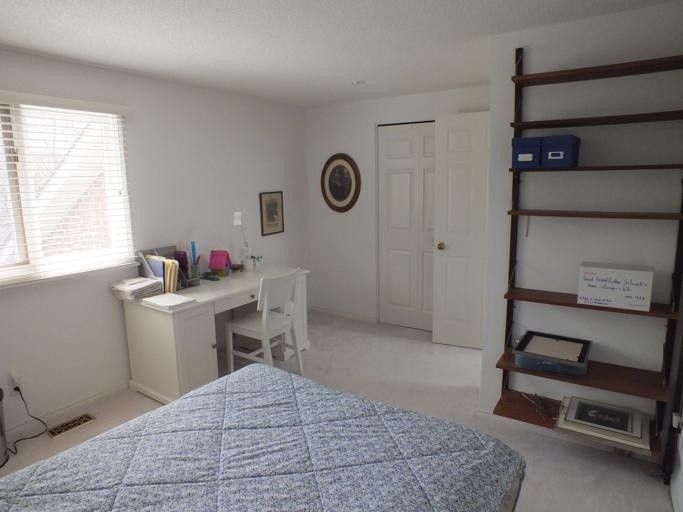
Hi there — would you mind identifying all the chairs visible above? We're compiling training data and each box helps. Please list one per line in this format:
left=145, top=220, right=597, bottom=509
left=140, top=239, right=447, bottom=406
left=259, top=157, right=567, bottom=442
left=225, top=267, right=306, bottom=380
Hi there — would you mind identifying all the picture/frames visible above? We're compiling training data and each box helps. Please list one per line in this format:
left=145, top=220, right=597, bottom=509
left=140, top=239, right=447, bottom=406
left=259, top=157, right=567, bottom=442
left=555, top=395, right=652, bottom=451
left=258, top=191, right=283, bottom=236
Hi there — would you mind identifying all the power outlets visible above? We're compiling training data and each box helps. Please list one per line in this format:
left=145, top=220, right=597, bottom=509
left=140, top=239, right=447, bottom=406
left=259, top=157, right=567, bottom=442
left=233, top=211, right=242, bottom=227
left=9, top=382, right=24, bottom=397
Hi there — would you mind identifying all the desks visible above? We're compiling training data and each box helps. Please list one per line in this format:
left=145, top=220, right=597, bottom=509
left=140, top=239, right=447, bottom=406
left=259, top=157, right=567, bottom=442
left=120, top=262, right=313, bottom=406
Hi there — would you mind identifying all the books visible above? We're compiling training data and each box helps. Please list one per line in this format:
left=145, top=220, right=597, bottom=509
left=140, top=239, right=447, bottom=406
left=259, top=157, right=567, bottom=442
left=111, top=255, right=197, bottom=314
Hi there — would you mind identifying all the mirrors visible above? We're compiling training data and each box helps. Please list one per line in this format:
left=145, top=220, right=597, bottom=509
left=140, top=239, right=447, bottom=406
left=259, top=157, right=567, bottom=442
left=320, top=153, right=361, bottom=213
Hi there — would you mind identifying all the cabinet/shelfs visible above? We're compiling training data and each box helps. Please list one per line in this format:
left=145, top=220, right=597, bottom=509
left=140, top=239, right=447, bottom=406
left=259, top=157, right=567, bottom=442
left=491, top=48, right=682, bottom=466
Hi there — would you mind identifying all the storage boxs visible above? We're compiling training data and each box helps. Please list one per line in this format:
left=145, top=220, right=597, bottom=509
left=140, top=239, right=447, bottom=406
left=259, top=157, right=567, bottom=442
left=510, top=135, right=540, bottom=169
left=574, top=260, right=655, bottom=314
left=540, top=135, right=580, bottom=168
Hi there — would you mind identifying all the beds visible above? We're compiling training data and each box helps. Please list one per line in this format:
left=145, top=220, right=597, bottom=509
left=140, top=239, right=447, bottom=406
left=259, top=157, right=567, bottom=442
left=0, top=361, right=528, bottom=510
